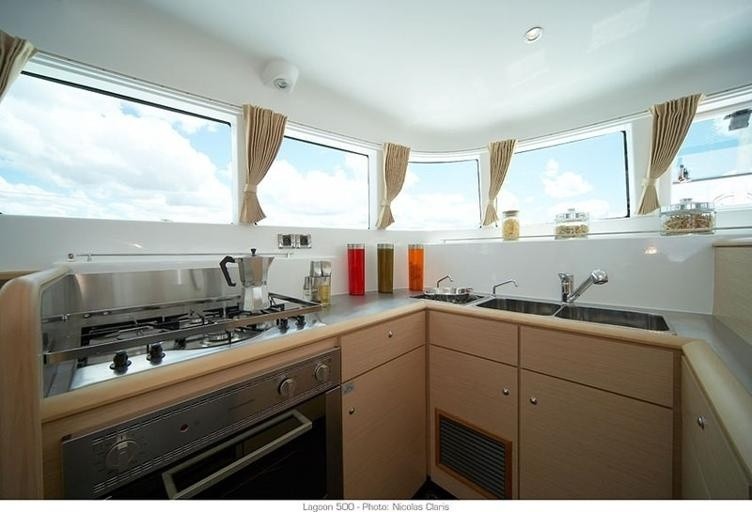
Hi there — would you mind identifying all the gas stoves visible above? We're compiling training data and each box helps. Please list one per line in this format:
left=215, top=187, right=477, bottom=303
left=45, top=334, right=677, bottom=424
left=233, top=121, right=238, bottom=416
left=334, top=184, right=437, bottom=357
left=41, top=288, right=325, bottom=395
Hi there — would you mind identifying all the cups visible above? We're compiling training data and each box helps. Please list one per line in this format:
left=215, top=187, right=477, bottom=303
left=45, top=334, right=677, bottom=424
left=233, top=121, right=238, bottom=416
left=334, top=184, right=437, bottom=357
left=303, top=260, right=332, bottom=305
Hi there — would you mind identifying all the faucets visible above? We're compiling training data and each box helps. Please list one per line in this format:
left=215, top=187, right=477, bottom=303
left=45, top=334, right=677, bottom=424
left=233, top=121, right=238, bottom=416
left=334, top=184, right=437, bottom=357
left=556, top=271, right=608, bottom=305
left=490, top=279, right=519, bottom=295
left=435, top=274, right=455, bottom=286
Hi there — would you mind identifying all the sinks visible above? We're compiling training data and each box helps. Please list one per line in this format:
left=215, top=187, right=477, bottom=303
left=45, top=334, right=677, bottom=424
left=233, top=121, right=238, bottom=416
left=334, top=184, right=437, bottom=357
left=477, top=295, right=564, bottom=316
left=554, top=303, right=678, bottom=336
left=409, top=294, right=488, bottom=305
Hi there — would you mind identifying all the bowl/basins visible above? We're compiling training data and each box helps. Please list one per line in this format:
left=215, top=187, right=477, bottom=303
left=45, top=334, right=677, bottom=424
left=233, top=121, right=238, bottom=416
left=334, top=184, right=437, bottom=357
left=423, top=285, right=475, bottom=301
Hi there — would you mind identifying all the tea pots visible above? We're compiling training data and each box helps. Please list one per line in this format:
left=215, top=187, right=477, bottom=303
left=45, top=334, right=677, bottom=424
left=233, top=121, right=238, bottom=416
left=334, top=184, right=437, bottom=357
left=219, top=248, right=276, bottom=313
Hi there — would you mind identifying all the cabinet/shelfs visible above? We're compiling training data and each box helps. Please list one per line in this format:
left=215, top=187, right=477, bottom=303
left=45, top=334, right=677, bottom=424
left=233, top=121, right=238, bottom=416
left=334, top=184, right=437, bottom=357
left=338, top=307, right=428, bottom=500
left=675, top=352, right=752, bottom=500
left=430, top=311, right=680, bottom=499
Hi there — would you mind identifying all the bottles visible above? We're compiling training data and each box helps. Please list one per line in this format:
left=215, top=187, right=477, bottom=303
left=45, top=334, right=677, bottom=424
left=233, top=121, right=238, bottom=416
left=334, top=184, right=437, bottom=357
left=409, top=244, right=424, bottom=291
left=660, top=198, right=717, bottom=238
left=555, top=209, right=589, bottom=241
left=502, top=210, right=520, bottom=240
left=348, top=244, right=365, bottom=296
left=377, top=244, right=394, bottom=293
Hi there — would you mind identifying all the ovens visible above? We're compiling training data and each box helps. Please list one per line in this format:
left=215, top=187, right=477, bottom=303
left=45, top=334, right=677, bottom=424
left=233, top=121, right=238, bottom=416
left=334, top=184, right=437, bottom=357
left=42, top=348, right=344, bottom=500
